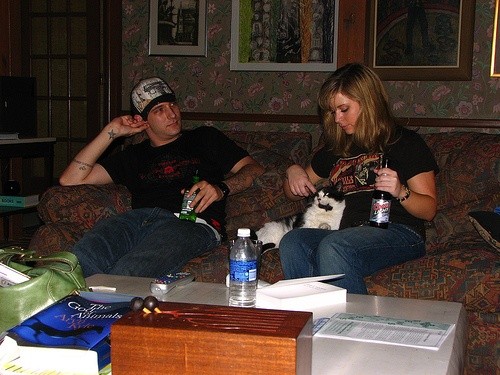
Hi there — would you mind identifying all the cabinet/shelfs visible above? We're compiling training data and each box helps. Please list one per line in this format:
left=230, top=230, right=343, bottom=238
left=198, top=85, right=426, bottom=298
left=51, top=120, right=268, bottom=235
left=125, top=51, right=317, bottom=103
left=0, top=137, right=56, bottom=241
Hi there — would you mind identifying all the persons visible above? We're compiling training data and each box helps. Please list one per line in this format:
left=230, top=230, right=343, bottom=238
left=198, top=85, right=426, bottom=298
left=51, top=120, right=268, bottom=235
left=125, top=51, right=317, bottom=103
left=279, top=62, right=438, bottom=295
left=58, top=76, right=266, bottom=279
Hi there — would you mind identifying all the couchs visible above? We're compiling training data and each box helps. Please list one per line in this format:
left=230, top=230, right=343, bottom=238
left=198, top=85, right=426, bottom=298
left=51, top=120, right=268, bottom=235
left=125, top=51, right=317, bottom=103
left=29, top=131, right=500, bottom=375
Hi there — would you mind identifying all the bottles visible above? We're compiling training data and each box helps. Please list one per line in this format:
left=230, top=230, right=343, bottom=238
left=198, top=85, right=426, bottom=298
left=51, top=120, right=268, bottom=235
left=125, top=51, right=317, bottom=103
left=179, top=168, right=201, bottom=223
left=368, top=155, right=393, bottom=229
left=228, top=227, right=257, bottom=309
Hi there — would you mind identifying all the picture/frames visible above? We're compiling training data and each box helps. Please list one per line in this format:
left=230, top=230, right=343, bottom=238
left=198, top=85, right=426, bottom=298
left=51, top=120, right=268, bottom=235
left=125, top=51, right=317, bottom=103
left=364, top=0, right=475, bottom=80
left=148, top=0, right=208, bottom=58
left=230, top=0, right=339, bottom=71
left=490, top=0, right=500, bottom=78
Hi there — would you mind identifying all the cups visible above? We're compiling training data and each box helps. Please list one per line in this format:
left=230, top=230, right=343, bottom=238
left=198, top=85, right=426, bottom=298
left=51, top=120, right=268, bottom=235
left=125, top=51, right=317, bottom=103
left=7, top=179, right=16, bottom=193
left=228, top=239, right=263, bottom=290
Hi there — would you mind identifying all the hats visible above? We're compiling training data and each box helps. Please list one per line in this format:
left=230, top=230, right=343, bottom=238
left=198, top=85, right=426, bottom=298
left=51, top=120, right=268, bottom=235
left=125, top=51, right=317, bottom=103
left=130, top=76, right=177, bottom=119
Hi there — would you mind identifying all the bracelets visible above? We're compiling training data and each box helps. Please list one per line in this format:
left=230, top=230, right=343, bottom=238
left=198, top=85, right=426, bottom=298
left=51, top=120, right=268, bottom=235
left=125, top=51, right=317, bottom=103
left=396, top=186, right=410, bottom=202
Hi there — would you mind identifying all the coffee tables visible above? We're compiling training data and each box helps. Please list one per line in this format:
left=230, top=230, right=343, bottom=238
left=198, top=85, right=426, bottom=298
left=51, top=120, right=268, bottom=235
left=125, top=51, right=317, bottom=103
left=84, top=274, right=469, bottom=375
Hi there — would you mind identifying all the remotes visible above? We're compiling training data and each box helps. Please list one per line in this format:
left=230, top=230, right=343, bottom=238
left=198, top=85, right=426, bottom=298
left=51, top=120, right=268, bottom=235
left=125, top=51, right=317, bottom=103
left=150, top=270, right=195, bottom=294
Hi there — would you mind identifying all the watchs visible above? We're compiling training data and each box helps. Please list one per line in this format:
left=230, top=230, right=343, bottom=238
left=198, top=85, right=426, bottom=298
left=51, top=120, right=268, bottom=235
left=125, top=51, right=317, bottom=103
left=215, top=183, right=230, bottom=200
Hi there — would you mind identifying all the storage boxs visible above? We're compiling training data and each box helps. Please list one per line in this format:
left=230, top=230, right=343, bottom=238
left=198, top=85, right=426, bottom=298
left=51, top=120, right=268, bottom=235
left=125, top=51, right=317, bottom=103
left=110, top=301, right=313, bottom=375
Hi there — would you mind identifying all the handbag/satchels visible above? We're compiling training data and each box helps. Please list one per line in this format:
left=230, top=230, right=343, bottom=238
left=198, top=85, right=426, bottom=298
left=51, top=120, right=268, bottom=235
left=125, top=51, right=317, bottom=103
left=0, top=246, right=90, bottom=337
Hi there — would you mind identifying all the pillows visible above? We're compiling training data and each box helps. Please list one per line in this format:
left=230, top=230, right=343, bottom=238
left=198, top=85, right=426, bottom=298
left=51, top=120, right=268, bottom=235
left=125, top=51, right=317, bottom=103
left=468, top=211, right=500, bottom=252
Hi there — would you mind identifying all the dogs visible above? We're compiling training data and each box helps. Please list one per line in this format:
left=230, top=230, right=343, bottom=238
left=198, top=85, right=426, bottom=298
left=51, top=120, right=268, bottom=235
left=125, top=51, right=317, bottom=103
left=229, top=180, right=346, bottom=257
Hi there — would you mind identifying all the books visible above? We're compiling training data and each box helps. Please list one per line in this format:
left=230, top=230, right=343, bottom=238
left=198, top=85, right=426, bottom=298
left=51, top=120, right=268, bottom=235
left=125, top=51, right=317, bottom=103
left=0, top=192, right=40, bottom=208
left=0, top=132, right=20, bottom=140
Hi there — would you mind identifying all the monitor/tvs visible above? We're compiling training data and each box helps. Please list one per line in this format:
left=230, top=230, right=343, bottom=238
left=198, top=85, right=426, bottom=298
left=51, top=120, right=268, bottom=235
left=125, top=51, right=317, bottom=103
left=0, top=75, right=38, bottom=139
